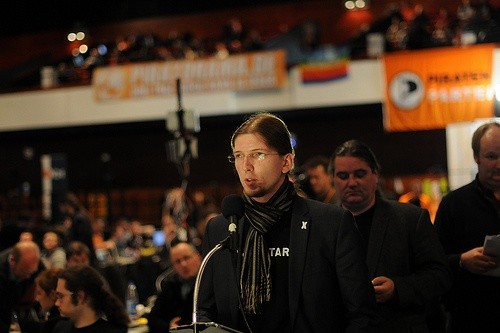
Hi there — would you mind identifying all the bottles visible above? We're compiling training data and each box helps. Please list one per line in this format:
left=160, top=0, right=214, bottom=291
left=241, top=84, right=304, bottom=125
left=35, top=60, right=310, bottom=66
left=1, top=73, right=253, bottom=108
left=126, top=283, right=137, bottom=318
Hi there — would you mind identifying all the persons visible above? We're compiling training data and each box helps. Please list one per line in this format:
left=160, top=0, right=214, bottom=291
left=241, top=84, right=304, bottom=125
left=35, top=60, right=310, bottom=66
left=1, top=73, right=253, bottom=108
left=0, top=185, right=236, bottom=333
left=294, top=158, right=339, bottom=206
left=192, top=113, right=381, bottom=333
left=433, top=123, right=500, bottom=333
left=57, top=0, right=500, bottom=84
left=333, top=139, right=453, bottom=333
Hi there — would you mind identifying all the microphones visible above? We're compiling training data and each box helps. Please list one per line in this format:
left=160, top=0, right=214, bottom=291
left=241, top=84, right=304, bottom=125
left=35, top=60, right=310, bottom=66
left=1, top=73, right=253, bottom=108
left=190, top=195, right=246, bottom=333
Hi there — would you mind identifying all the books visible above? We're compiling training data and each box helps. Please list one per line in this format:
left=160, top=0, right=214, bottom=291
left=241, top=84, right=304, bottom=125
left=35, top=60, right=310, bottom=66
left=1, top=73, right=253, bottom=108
left=476, top=234, right=500, bottom=276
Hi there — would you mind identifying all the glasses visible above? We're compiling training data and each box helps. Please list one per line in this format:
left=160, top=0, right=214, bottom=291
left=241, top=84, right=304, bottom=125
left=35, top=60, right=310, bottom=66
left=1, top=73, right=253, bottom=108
left=227, top=151, right=284, bottom=164
